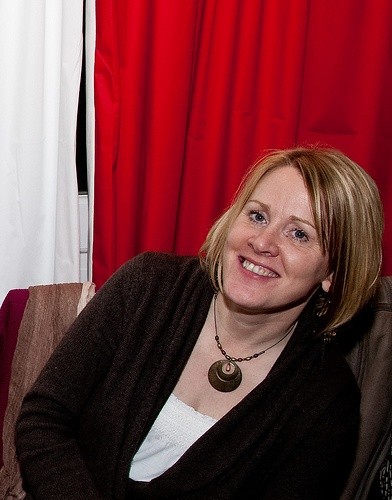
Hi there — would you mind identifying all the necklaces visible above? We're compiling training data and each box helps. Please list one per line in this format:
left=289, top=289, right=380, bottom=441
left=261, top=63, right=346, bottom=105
left=207, top=291, right=297, bottom=393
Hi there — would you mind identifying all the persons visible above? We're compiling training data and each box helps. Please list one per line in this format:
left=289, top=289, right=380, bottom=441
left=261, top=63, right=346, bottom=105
left=14, top=147, right=383, bottom=500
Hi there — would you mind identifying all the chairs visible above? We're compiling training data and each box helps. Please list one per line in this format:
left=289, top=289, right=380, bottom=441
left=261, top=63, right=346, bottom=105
left=1, top=282, right=391, bottom=499
